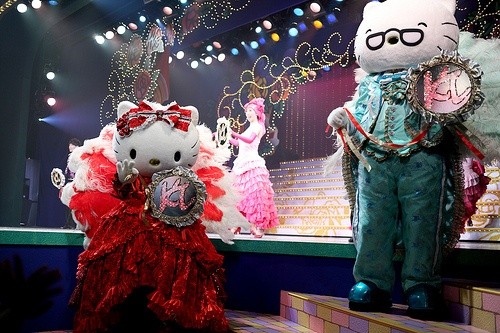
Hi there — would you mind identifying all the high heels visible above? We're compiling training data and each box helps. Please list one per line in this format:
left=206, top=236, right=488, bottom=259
left=234, top=227, right=241, bottom=234
left=252, top=231, right=262, bottom=238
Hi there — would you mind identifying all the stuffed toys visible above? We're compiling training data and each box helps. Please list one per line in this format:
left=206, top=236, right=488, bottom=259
left=326, top=1, right=483, bottom=316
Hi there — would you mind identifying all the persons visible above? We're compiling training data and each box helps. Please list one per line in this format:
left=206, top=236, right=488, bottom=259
left=58, top=139, right=87, bottom=228
left=221, top=97, right=279, bottom=240
left=108, top=98, right=230, bottom=333
